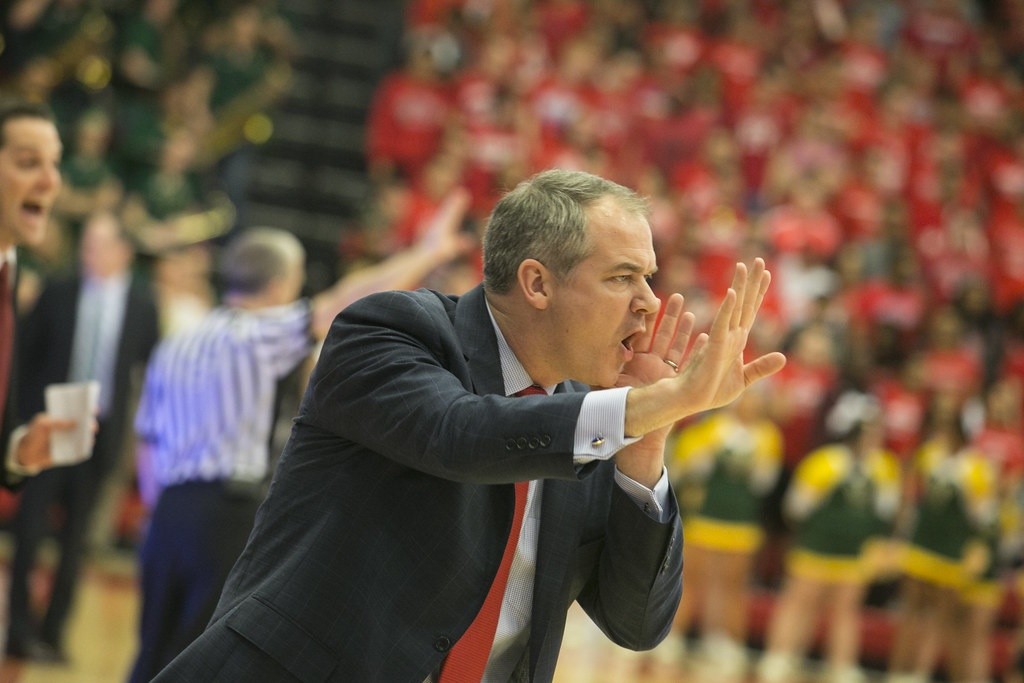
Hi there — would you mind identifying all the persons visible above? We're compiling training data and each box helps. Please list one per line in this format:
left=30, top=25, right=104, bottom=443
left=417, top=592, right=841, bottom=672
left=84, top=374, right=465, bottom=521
left=0, top=0, right=1024, bottom=666
left=128, top=188, right=474, bottom=683
left=149, top=171, right=787, bottom=683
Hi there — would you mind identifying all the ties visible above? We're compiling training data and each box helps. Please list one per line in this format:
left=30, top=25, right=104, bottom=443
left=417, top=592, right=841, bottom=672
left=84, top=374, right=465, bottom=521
left=436, top=386, right=549, bottom=683
left=0, top=261, right=15, bottom=427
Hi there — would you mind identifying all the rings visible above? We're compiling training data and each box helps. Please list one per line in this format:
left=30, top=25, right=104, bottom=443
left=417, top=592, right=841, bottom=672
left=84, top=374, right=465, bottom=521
left=663, top=358, right=679, bottom=372
